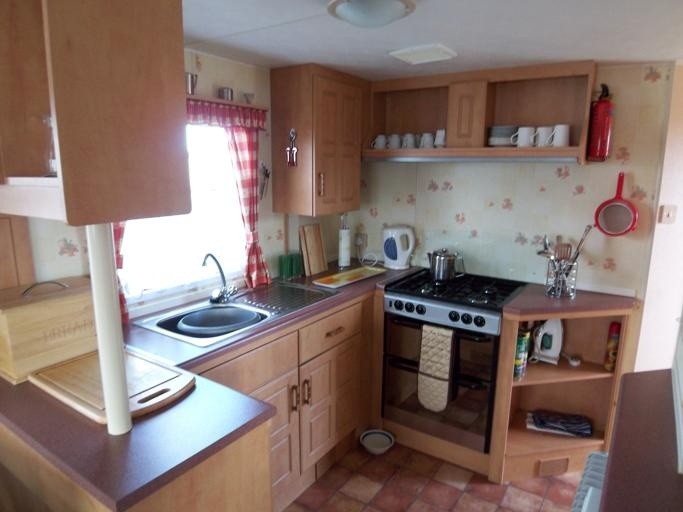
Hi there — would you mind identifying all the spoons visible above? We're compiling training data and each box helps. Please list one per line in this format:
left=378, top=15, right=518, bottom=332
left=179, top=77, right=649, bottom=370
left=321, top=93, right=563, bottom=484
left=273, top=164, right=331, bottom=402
left=536, top=235, right=558, bottom=298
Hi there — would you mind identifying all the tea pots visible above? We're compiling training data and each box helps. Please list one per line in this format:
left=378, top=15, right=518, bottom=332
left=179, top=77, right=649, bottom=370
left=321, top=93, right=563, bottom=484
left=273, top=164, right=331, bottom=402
left=425, top=247, right=467, bottom=287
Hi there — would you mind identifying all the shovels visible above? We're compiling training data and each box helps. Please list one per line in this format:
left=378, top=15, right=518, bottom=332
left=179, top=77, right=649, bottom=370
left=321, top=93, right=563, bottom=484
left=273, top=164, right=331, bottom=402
left=554, top=243, right=572, bottom=265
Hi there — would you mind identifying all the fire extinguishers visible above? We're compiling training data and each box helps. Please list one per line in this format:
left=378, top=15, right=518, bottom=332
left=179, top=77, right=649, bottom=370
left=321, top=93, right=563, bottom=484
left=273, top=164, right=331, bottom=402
left=586, top=83, right=617, bottom=165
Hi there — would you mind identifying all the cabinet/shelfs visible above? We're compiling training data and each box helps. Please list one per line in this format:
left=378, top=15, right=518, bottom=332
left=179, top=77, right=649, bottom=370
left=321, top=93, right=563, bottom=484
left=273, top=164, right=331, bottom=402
left=266, top=61, right=370, bottom=218
left=488, top=277, right=643, bottom=487
left=196, top=298, right=376, bottom=512
left=357, top=58, right=598, bottom=166
left=0, top=1, right=200, bottom=228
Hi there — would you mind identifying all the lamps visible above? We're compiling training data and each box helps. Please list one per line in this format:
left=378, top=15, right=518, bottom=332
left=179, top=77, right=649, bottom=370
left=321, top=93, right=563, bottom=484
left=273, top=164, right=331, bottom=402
left=324, top=0, right=419, bottom=30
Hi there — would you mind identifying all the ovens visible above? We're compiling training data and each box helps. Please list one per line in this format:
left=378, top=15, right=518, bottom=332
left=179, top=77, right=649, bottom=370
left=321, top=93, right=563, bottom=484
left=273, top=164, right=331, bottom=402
left=380, top=312, right=501, bottom=455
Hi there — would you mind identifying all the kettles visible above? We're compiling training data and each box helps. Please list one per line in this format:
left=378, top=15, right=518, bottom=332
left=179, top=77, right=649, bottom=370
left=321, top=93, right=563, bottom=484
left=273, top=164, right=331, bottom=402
left=338, top=221, right=351, bottom=270
left=380, top=225, right=415, bottom=270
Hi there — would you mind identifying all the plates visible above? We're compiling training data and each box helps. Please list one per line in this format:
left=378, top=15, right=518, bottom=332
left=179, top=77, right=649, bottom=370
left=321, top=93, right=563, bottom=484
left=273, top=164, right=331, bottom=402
left=486, top=123, right=520, bottom=146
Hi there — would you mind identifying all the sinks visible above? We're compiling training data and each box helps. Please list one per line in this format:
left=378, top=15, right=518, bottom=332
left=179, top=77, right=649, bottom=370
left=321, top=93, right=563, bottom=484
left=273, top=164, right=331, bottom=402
left=132, top=300, right=274, bottom=349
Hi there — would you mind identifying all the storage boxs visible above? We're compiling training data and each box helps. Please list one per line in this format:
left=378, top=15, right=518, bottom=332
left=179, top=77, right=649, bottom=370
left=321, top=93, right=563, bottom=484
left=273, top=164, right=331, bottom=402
left=0, top=267, right=100, bottom=388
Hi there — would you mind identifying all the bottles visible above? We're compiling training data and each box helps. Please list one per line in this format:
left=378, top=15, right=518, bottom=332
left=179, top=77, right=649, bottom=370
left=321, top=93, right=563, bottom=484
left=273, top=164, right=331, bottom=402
left=513, top=322, right=534, bottom=381
left=603, top=321, right=619, bottom=371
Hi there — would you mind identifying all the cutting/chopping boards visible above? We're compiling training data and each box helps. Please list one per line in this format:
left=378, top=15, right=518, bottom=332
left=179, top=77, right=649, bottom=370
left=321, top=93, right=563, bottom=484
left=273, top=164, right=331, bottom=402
left=25, top=346, right=196, bottom=426
left=298, top=222, right=329, bottom=276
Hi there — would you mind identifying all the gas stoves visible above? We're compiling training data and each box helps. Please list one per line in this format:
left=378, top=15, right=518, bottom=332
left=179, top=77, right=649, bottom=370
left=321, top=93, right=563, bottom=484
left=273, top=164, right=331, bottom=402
left=375, top=265, right=528, bottom=336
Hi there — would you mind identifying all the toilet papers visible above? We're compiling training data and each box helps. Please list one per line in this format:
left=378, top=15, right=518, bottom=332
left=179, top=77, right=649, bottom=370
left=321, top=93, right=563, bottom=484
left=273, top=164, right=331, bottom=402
left=337, top=229, right=352, bottom=268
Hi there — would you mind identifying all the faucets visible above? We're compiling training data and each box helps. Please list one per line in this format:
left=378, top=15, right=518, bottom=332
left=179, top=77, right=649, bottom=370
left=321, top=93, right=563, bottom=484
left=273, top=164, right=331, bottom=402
left=202, top=254, right=238, bottom=304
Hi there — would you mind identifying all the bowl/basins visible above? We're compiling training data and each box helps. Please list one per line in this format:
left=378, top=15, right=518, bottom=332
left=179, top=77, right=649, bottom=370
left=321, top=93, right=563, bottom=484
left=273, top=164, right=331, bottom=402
left=358, top=427, right=396, bottom=457
left=172, top=304, right=260, bottom=336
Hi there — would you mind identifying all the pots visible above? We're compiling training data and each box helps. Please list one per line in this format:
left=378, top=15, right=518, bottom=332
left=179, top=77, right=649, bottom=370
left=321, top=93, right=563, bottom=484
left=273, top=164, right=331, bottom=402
left=591, top=172, right=641, bottom=238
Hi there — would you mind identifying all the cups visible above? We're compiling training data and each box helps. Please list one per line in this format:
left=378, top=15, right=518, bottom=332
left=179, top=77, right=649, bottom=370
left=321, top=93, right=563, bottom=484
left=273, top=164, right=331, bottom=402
left=243, top=91, right=257, bottom=105
left=290, top=252, right=305, bottom=278
left=217, top=86, right=234, bottom=102
left=369, top=127, right=447, bottom=151
left=278, top=254, right=290, bottom=279
left=186, top=71, right=198, bottom=95
left=545, top=258, right=579, bottom=302
left=509, top=123, right=570, bottom=148
